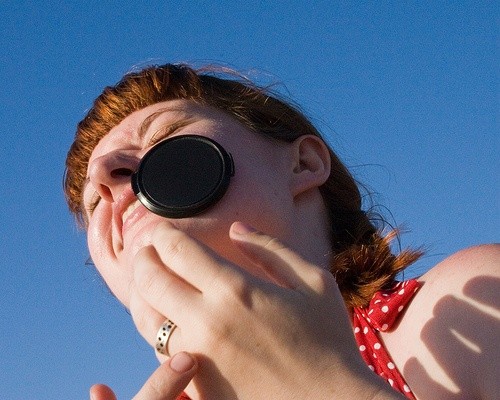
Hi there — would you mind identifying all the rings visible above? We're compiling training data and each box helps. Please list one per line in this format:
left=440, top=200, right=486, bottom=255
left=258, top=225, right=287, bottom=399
left=156, top=318, right=177, bottom=356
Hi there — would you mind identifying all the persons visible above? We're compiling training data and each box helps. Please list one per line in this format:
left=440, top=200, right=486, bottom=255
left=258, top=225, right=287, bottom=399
left=62, top=62, right=499, bottom=400
